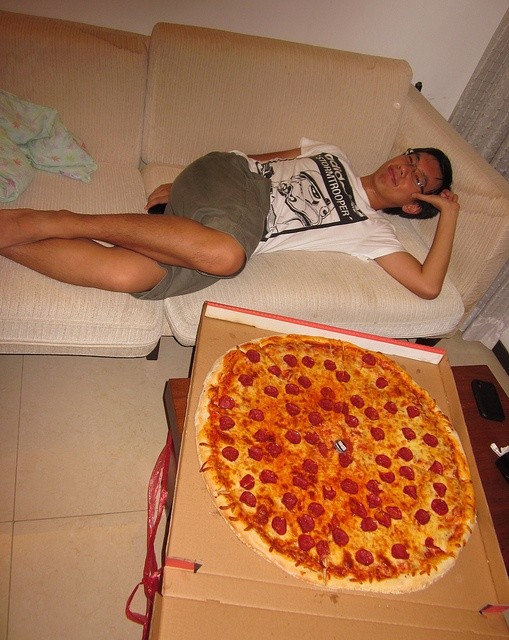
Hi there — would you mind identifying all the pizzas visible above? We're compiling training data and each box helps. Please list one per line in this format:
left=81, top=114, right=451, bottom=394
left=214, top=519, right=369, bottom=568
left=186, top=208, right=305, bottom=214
left=195, top=334, right=477, bottom=592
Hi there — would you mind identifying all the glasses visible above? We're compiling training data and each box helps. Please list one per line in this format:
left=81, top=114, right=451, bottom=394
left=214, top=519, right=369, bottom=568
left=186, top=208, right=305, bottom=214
left=403, top=148, right=427, bottom=195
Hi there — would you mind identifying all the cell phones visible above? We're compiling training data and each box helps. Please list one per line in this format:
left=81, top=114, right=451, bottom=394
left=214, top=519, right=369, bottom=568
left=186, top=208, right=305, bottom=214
left=470, top=378, right=505, bottom=424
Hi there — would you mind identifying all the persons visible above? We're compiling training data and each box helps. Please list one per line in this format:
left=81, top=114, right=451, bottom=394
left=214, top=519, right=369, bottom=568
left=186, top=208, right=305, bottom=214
left=0, top=136, right=461, bottom=301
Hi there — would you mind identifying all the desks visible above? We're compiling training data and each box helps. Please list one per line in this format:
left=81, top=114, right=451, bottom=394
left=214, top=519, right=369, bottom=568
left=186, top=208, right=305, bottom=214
left=165, top=366, right=509, bottom=640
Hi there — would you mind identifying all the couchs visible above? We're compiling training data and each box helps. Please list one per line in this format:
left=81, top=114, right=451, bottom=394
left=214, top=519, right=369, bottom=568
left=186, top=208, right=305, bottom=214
left=143, top=12, right=509, bottom=345
left=0, top=8, right=165, bottom=362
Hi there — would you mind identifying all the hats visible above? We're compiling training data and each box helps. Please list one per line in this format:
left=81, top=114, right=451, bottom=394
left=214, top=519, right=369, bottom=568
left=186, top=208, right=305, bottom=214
left=302, top=171, right=334, bottom=211
